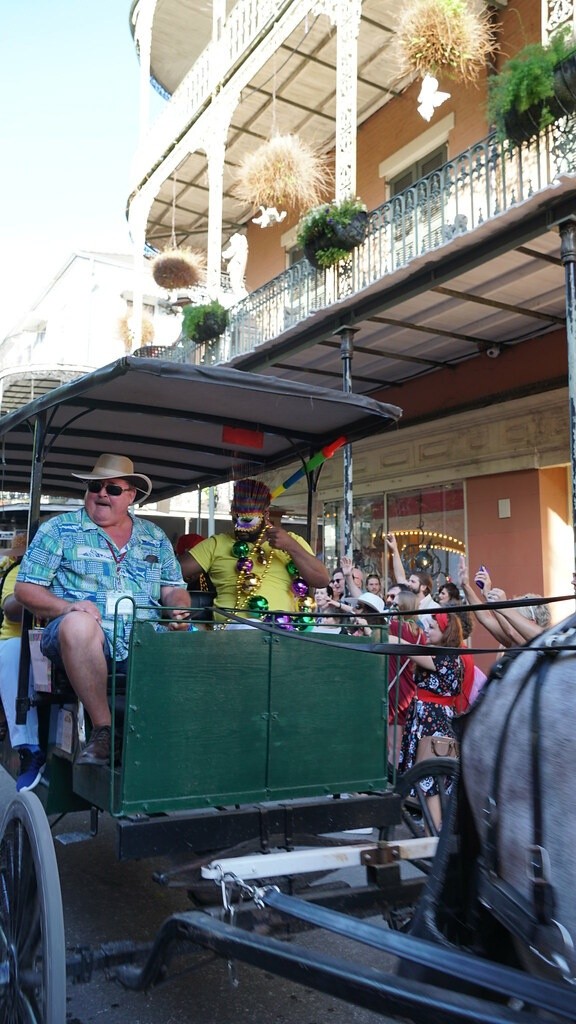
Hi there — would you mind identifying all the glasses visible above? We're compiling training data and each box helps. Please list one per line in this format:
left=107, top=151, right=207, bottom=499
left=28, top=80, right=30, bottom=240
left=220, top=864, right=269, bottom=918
left=86, top=481, right=132, bottom=496
left=387, top=594, right=396, bottom=599
left=390, top=602, right=399, bottom=607
left=331, top=579, right=341, bottom=583
left=355, top=604, right=365, bottom=611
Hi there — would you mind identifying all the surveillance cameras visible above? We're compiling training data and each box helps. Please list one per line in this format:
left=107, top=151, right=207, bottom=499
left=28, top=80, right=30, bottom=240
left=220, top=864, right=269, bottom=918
left=487, top=346, right=501, bottom=358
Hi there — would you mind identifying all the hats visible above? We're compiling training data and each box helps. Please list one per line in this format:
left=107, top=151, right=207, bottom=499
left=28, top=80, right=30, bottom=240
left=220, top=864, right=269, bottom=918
left=177, top=534, right=206, bottom=554
left=71, top=453, right=152, bottom=506
left=344, top=592, right=388, bottom=625
left=0, top=533, right=26, bottom=556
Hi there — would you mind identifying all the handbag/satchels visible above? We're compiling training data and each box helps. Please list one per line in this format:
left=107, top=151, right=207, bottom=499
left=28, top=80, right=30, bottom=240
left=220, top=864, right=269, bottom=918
left=415, top=734, right=457, bottom=763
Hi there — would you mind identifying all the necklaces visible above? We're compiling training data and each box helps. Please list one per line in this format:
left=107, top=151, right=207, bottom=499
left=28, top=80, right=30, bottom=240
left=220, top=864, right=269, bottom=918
left=217, top=524, right=275, bottom=628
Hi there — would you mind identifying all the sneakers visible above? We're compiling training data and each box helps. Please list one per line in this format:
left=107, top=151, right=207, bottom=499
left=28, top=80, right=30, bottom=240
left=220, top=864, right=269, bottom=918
left=16, top=748, right=46, bottom=793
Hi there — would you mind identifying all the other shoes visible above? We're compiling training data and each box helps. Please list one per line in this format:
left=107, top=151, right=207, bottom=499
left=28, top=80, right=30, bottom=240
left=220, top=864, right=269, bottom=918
left=342, top=827, right=374, bottom=834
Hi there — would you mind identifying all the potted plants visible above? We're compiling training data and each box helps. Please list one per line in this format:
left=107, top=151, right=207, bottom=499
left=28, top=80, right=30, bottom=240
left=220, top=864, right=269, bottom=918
left=182, top=299, right=227, bottom=344
left=484, top=24, right=576, bottom=148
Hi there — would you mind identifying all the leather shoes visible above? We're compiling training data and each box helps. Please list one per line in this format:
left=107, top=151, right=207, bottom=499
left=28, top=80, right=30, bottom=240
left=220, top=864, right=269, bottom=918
left=77, top=725, right=120, bottom=766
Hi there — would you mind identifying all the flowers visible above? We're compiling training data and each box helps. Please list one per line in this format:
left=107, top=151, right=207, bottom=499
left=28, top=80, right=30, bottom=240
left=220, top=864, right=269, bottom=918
left=298, top=196, right=367, bottom=250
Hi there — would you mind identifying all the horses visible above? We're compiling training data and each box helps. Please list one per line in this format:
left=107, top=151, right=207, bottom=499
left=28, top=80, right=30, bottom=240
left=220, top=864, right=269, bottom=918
left=457, top=612, right=576, bottom=1024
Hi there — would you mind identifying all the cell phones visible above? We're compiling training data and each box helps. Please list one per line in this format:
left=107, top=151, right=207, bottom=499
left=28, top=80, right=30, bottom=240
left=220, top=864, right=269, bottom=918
left=476, top=567, right=489, bottom=589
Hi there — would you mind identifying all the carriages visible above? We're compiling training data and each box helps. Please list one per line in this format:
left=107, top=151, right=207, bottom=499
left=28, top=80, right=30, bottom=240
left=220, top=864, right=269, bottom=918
left=0, top=356, right=576, bottom=1023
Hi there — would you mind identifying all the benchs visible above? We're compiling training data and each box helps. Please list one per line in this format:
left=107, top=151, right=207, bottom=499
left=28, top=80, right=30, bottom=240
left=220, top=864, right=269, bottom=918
left=32, top=589, right=216, bottom=755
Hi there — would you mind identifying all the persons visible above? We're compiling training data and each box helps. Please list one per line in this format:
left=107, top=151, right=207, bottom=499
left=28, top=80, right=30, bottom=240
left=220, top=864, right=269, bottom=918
left=14, top=456, right=192, bottom=765
left=0, top=521, right=46, bottom=792
left=177, top=534, right=206, bottom=591
left=316, top=533, right=576, bottom=837
left=179, top=479, right=330, bottom=629
